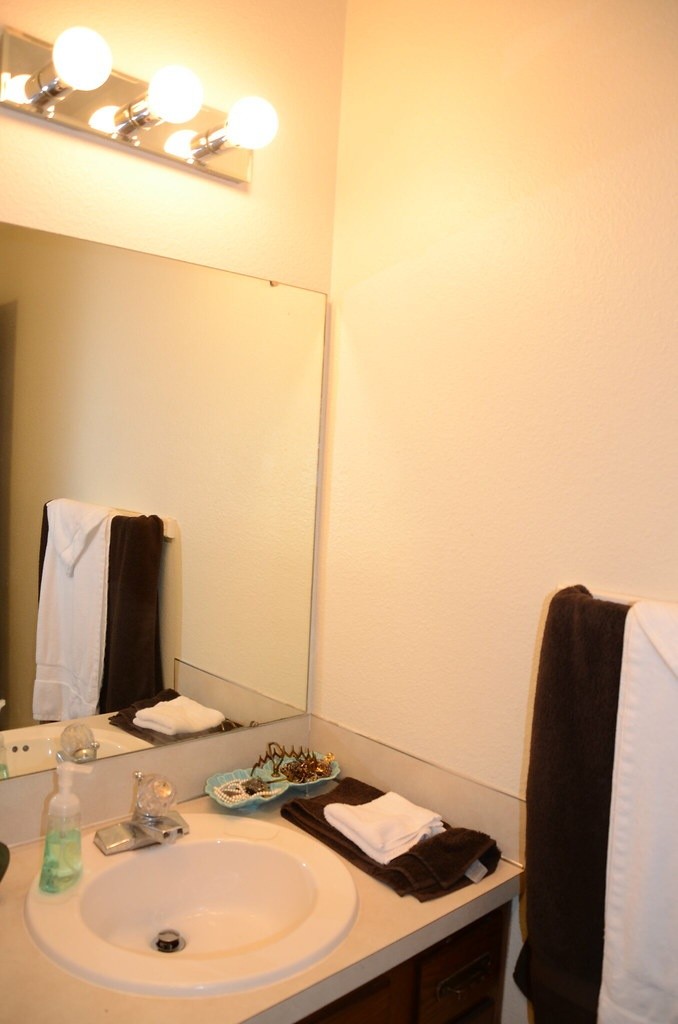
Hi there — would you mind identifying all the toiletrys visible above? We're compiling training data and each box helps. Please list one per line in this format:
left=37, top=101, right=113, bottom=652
left=0, top=698, right=10, bottom=781
left=38, top=761, right=94, bottom=894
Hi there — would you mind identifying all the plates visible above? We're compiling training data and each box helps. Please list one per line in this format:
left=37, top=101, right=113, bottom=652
left=205, top=751, right=340, bottom=809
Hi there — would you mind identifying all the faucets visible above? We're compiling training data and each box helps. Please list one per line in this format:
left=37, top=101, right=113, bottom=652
left=92, top=770, right=191, bottom=856
left=54, top=723, right=101, bottom=764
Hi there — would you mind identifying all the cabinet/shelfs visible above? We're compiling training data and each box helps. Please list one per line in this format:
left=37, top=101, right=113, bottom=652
left=295, top=899, right=513, bottom=1024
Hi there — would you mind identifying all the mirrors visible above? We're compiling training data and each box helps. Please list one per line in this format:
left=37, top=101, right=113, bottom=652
left=0, top=222, right=327, bottom=780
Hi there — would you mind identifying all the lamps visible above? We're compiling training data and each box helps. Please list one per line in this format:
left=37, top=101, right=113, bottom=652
left=39, top=26, right=112, bottom=102
left=3, top=74, right=56, bottom=115
left=208, top=96, right=278, bottom=154
left=130, top=67, right=203, bottom=130
left=88, top=105, right=139, bottom=141
left=164, top=130, right=211, bottom=163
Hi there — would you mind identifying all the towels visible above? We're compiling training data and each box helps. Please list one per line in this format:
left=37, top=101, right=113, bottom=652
left=280, top=776, right=502, bottom=903
left=108, top=688, right=227, bottom=747
left=30, top=498, right=165, bottom=724
left=510, top=585, right=677, bottom=1024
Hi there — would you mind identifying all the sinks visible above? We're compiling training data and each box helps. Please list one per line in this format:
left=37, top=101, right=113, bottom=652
left=23, top=811, right=359, bottom=999
left=0, top=725, right=154, bottom=781
